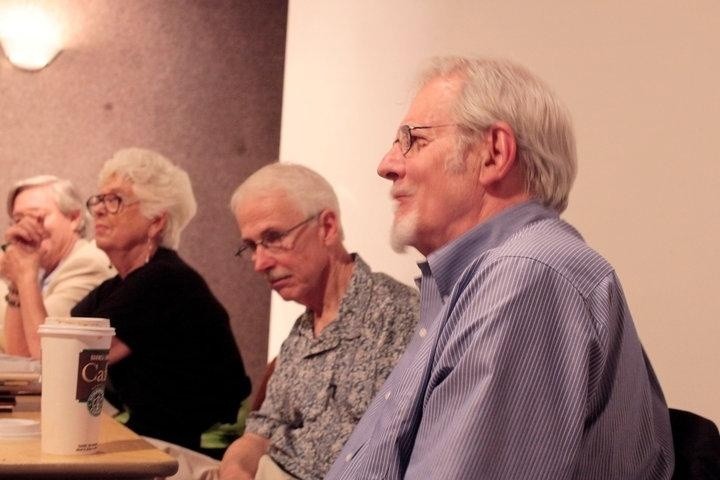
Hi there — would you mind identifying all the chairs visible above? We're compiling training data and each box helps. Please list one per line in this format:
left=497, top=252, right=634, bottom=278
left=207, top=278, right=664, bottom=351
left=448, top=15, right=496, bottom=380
left=667, top=407, right=720, bottom=480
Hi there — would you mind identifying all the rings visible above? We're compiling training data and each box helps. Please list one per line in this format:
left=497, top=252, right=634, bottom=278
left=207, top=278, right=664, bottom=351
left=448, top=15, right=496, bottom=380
left=2, top=242, right=11, bottom=251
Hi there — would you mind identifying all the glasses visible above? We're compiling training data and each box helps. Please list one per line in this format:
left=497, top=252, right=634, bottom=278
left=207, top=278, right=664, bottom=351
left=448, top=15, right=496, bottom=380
left=235, top=216, right=315, bottom=262
left=87, top=193, right=121, bottom=217
left=393, top=124, right=460, bottom=155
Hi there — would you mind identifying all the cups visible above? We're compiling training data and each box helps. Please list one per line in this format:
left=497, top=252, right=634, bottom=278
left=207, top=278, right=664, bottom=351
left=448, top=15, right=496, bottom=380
left=37, top=317, right=117, bottom=456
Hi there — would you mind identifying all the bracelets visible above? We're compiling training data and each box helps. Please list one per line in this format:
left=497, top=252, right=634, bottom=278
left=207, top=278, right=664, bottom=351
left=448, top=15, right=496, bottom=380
left=7, top=286, right=19, bottom=295
left=5, top=295, right=21, bottom=307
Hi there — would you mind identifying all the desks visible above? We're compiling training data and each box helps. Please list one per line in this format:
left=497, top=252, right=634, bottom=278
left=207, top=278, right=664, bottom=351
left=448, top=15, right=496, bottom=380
left=1, top=387, right=179, bottom=480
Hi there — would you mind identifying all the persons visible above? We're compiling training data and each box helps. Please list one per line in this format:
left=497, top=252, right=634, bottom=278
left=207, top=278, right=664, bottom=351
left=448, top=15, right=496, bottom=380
left=0, top=146, right=252, bottom=462
left=0, top=175, right=118, bottom=361
left=139, top=161, right=420, bottom=480
left=323, top=57, right=675, bottom=480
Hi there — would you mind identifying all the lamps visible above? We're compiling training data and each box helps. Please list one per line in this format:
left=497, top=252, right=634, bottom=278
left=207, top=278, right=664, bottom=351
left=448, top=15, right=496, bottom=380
left=0, top=4, right=64, bottom=71
